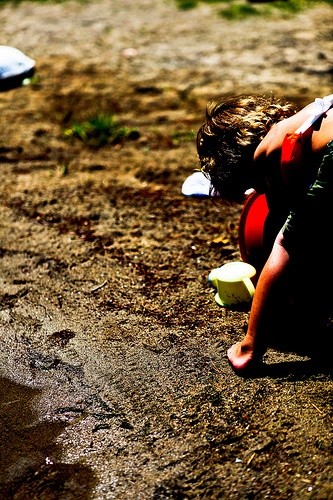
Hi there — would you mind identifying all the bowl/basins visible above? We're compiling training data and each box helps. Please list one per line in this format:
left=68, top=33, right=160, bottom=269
left=237, top=190, right=278, bottom=274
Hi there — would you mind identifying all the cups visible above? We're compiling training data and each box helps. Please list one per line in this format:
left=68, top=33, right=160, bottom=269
left=213, top=261, right=257, bottom=305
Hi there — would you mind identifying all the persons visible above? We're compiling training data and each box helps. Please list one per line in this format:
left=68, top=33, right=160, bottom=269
left=194, top=92, right=332, bottom=370
